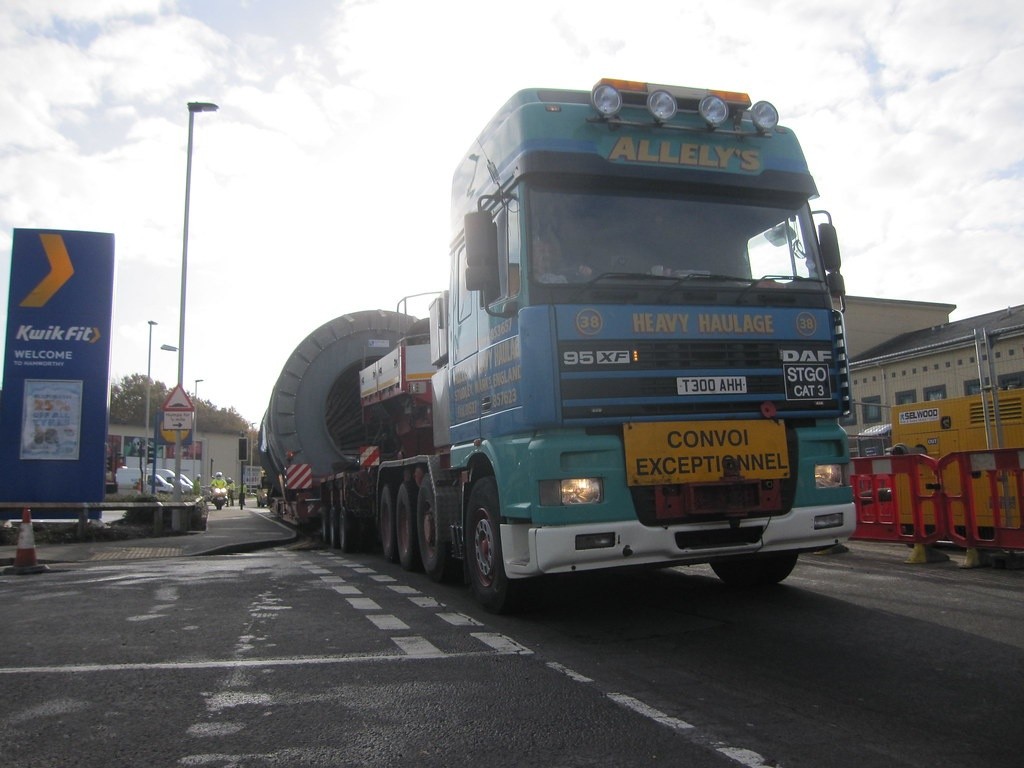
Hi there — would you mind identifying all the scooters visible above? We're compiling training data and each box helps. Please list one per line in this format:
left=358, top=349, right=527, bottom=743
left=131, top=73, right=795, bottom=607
left=210, top=486, right=229, bottom=510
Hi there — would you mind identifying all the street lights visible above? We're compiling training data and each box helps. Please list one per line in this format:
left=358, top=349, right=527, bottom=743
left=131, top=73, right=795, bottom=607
left=144, top=321, right=158, bottom=495
left=171, top=102, right=220, bottom=533
left=193, top=379, right=204, bottom=479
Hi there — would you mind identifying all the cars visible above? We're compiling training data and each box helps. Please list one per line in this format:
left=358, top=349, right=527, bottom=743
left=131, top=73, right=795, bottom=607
left=257, top=489, right=268, bottom=508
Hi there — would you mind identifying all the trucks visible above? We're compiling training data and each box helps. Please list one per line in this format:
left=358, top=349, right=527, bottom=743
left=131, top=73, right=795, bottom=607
left=117, top=474, right=174, bottom=496
left=116, top=468, right=193, bottom=499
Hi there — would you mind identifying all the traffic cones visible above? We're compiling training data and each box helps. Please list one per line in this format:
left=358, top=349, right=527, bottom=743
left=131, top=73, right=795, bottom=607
left=4, top=509, right=49, bottom=574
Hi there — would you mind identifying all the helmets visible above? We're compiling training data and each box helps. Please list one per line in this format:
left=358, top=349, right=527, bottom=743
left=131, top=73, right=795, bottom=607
left=216, top=472, right=222, bottom=478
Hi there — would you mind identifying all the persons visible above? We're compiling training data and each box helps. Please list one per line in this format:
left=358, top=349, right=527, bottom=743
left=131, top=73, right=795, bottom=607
left=209, top=472, right=228, bottom=503
left=192, top=473, right=202, bottom=496
left=237, top=481, right=248, bottom=505
left=226, top=477, right=235, bottom=506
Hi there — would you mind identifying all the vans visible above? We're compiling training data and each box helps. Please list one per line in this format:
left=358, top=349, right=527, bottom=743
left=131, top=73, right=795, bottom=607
left=180, top=474, right=193, bottom=487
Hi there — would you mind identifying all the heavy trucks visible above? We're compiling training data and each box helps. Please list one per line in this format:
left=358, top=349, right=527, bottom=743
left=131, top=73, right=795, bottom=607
left=256, top=77, right=856, bottom=616
